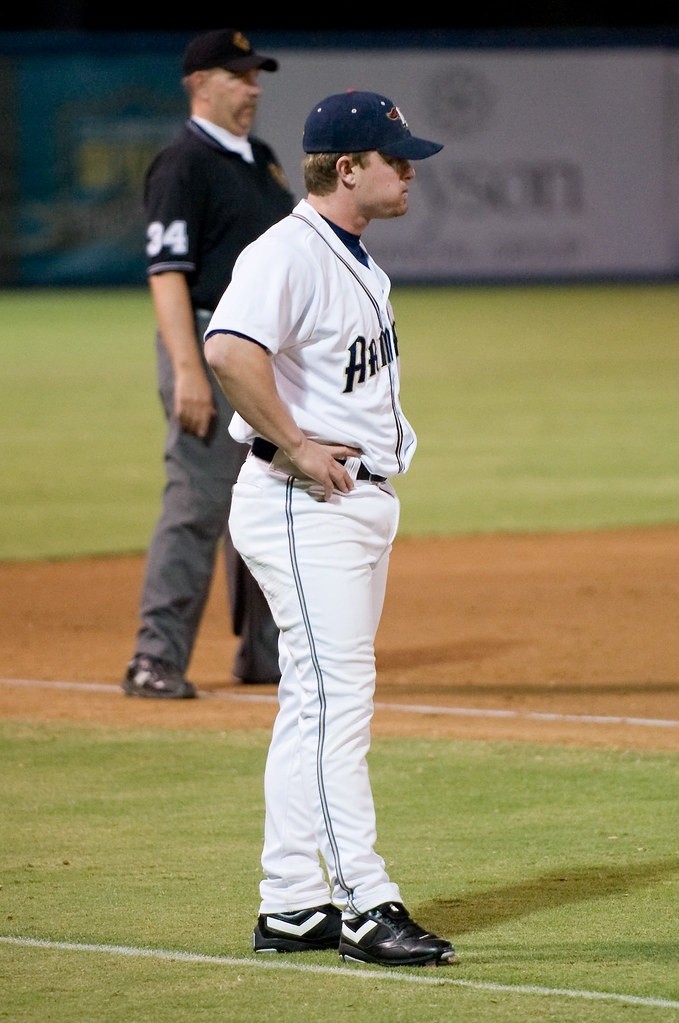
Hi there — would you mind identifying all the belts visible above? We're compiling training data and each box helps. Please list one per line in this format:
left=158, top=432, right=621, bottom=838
left=252, top=436, right=387, bottom=484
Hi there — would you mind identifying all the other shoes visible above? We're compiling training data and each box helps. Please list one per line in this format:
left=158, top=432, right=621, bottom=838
left=232, top=643, right=282, bottom=683
left=118, top=652, right=197, bottom=700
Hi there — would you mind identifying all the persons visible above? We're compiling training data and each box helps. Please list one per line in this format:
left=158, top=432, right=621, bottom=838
left=123, top=30, right=282, bottom=698
left=202, top=90, right=454, bottom=961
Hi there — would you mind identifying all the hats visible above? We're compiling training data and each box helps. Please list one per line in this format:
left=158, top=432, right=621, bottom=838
left=302, top=89, right=446, bottom=160
left=181, top=28, right=278, bottom=76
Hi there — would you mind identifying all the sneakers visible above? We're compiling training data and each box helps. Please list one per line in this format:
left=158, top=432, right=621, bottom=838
left=339, top=901, right=457, bottom=967
left=251, top=903, right=342, bottom=955
